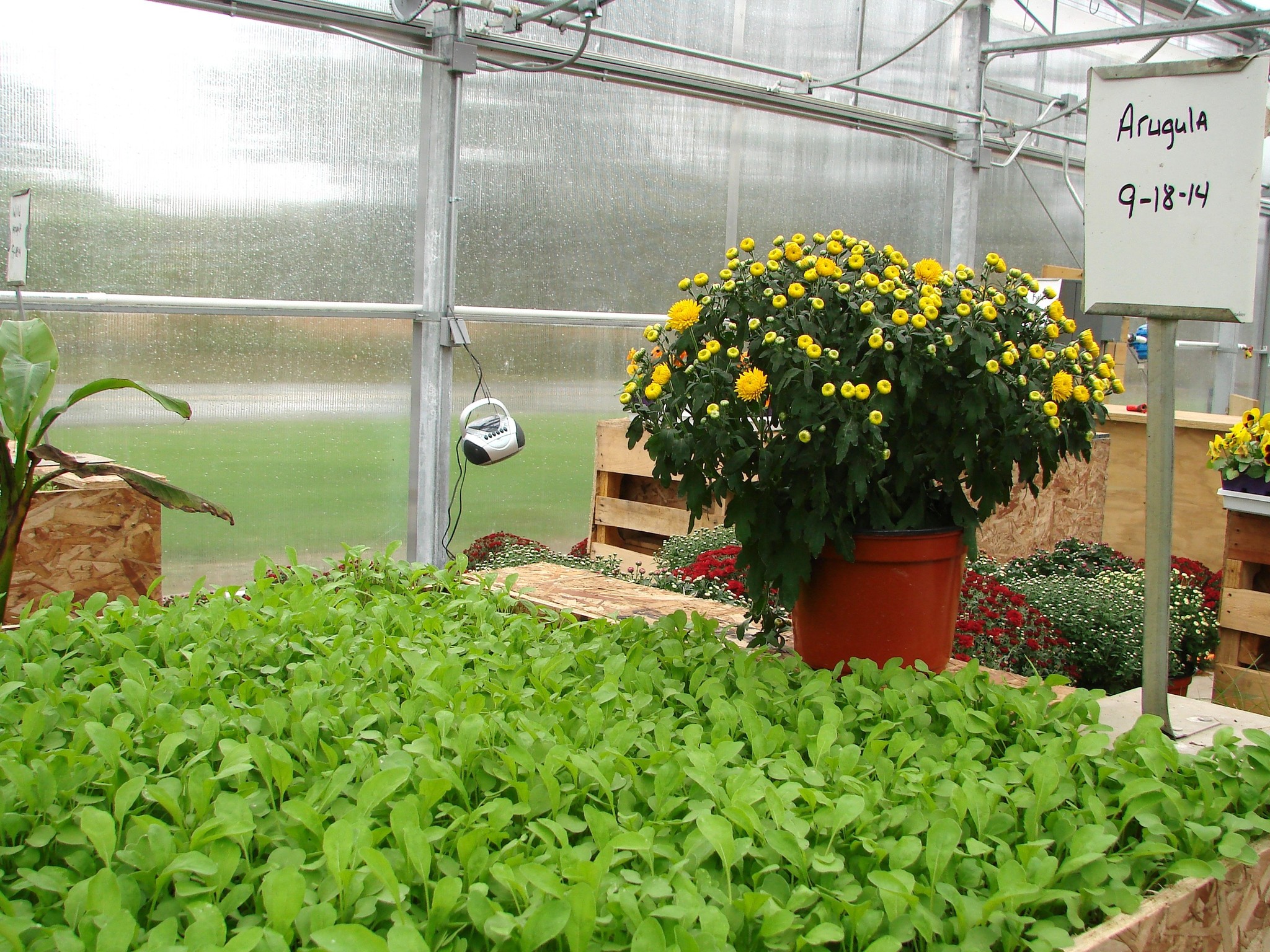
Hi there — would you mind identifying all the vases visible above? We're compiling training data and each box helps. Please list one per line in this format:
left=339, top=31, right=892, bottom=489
left=782, top=509, right=978, bottom=680
left=1217, top=465, right=1270, bottom=496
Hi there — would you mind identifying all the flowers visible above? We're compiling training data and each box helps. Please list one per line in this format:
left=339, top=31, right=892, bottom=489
left=465, top=228, right=1270, bottom=688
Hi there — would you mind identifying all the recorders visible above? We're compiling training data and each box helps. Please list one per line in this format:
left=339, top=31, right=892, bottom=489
left=459, top=398, right=525, bottom=466
